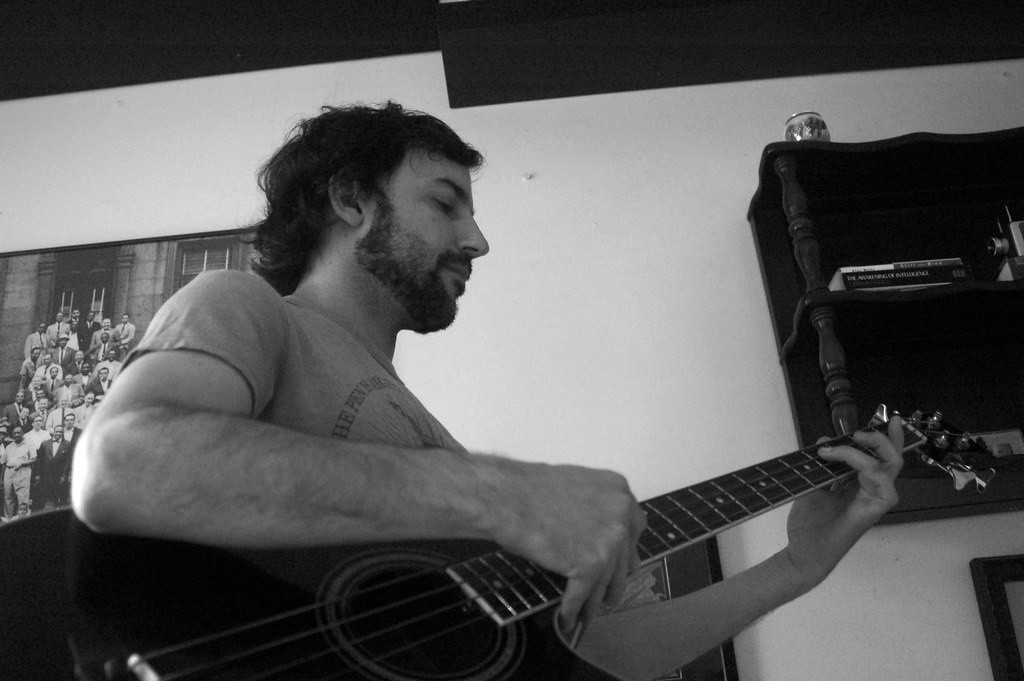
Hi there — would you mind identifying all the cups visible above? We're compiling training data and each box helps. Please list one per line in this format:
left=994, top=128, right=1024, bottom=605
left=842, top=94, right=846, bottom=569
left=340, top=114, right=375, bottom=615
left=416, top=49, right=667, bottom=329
left=784, top=112, right=830, bottom=141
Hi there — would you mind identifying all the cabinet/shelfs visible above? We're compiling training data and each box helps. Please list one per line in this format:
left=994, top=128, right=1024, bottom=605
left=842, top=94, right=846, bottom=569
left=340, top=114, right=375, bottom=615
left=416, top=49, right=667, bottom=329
left=746, top=129, right=1024, bottom=526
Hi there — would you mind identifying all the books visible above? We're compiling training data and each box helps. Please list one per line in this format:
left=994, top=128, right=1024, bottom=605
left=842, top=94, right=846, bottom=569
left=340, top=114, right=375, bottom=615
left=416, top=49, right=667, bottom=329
left=828, top=258, right=967, bottom=291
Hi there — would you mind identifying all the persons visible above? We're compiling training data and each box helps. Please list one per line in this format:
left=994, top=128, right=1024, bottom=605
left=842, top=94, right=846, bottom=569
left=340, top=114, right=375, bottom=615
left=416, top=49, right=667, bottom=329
left=71, top=99, right=904, bottom=681
left=0, top=306, right=136, bottom=522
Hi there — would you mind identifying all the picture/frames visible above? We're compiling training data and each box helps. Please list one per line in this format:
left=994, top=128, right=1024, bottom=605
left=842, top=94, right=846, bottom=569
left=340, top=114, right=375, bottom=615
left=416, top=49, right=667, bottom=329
left=650, top=534, right=739, bottom=681
left=0, top=227, right=254, bottom=529
left=969, top=553, right=1024, bottom=681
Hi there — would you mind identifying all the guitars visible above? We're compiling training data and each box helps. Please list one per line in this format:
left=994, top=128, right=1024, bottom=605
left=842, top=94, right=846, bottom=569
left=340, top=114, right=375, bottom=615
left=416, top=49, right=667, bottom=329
left=0, top=405, right=1001, bottom=681
